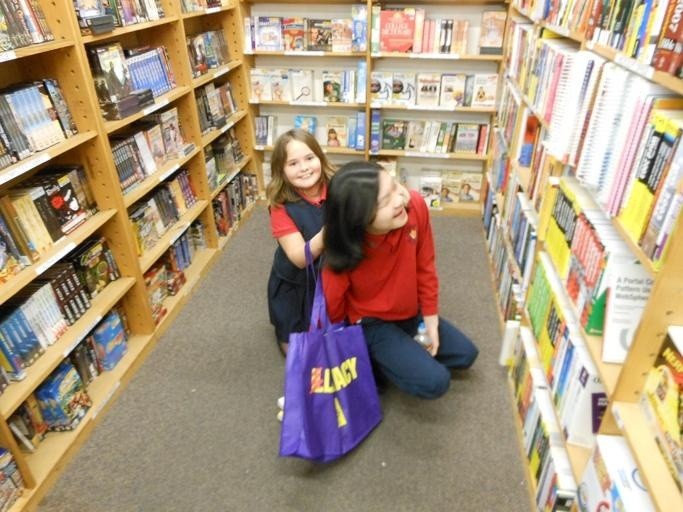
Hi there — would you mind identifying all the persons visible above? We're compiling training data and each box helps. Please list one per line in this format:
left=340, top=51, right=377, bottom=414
left=309, top=161, right=479, bottom=400
left=269, top=131, right=351, bottom=423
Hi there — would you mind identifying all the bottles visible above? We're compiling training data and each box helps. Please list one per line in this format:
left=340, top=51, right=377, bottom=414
left=414, top=322, right=433, bottom=355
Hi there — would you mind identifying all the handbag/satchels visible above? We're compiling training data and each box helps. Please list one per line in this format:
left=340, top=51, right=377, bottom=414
left=278, top=241, right=385, bottom=463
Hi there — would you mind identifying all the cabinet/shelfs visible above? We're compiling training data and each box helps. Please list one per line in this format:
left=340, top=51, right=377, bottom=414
left=478, top=0, right=683, bottom=512
left=0, top=0, right=269, bottom=512
left=221, top=0, right=512, bottom=219
